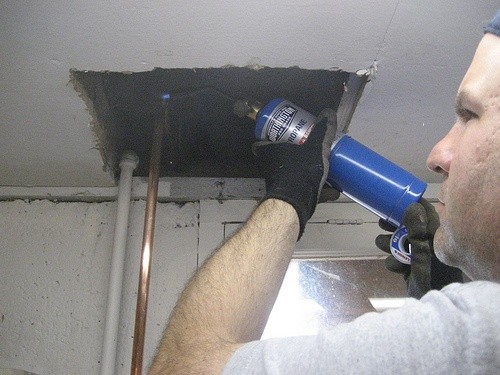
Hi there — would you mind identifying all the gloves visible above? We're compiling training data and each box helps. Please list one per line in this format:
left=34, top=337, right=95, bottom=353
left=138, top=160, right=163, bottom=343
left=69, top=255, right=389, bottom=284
left=375, top=194, right=465, bottom=300
left=249, top=109, right=339, bottom=240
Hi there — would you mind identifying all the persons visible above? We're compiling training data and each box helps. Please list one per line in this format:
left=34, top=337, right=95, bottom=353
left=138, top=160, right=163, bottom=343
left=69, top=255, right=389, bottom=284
left=147, top=11, right=499, bottom=374
